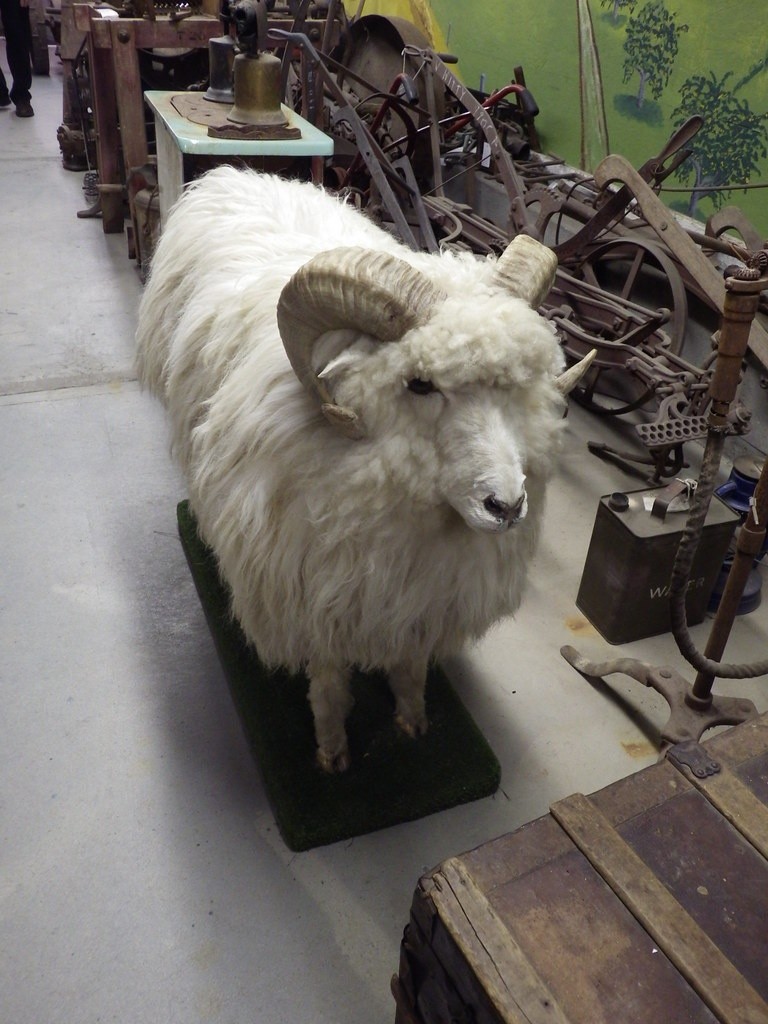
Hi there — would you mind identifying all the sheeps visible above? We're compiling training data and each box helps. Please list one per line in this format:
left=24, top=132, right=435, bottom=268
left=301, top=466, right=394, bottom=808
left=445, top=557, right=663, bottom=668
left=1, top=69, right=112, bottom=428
left=135, top=162, right=596, bottom=775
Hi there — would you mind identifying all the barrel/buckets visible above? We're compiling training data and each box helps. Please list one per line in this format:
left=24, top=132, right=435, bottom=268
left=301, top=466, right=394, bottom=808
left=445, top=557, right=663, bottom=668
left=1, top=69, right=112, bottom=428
left=574, top=477, right=740, bottom=646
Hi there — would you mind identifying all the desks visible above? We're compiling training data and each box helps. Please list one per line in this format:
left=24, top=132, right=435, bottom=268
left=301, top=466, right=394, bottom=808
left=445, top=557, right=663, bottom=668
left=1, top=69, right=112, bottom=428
left=142, top=89, right=334, bottom=236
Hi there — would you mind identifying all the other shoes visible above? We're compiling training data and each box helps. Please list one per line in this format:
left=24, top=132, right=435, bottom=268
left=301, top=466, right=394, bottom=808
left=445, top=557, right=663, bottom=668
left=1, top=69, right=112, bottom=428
left=0, top=94, right=11, bottom=108
left=16, top=102, right=34, bottom=117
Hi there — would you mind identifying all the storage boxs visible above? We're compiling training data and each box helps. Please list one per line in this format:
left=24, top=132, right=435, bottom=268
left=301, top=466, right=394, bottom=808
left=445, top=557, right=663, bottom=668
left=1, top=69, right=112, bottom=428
left=391, top=710, right=768, bottom=1024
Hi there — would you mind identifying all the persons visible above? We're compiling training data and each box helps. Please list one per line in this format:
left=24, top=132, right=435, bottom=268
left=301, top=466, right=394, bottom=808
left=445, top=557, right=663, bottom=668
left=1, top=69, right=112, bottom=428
left=0, top=0, right=34, bottom=117
